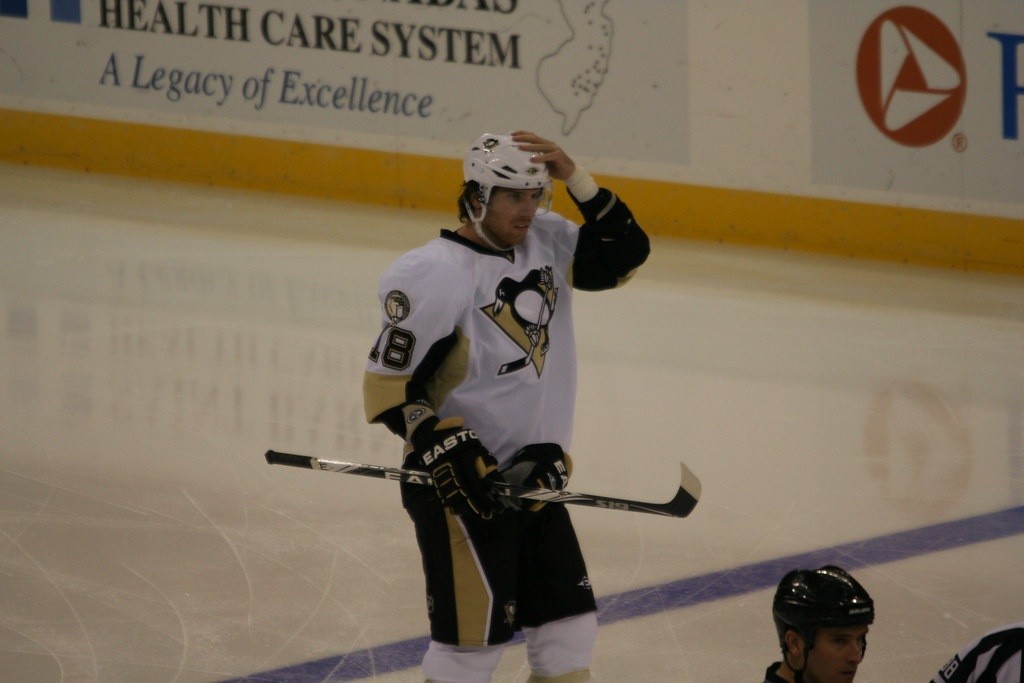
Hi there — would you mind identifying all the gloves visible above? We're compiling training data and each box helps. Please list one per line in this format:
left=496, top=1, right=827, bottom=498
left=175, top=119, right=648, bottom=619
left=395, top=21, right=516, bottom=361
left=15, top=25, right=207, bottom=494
left=411, top=416, right=496, bottom=519
left=495, top=443, right=573, bottom=514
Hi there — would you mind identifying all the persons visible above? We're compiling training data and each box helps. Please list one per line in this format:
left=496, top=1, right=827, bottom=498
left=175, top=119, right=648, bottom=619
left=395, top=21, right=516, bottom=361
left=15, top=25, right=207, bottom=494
left=362, top=129, right=653, bottom=683
left=928, top=619, right=1024, bottom=683
left=763, top=565, right=875, bottom=683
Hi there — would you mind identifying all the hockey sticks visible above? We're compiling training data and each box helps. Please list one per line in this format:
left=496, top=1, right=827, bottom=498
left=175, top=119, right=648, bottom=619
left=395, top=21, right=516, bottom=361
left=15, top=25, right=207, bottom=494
left=265, top=449, right=702, bottom=520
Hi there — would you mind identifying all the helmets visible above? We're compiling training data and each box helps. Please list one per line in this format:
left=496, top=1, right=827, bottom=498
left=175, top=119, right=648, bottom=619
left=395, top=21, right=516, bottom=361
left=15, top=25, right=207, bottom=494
left=773, top=565, right=875, bottom=649
left=463, top=133, right=553, bottom=204
